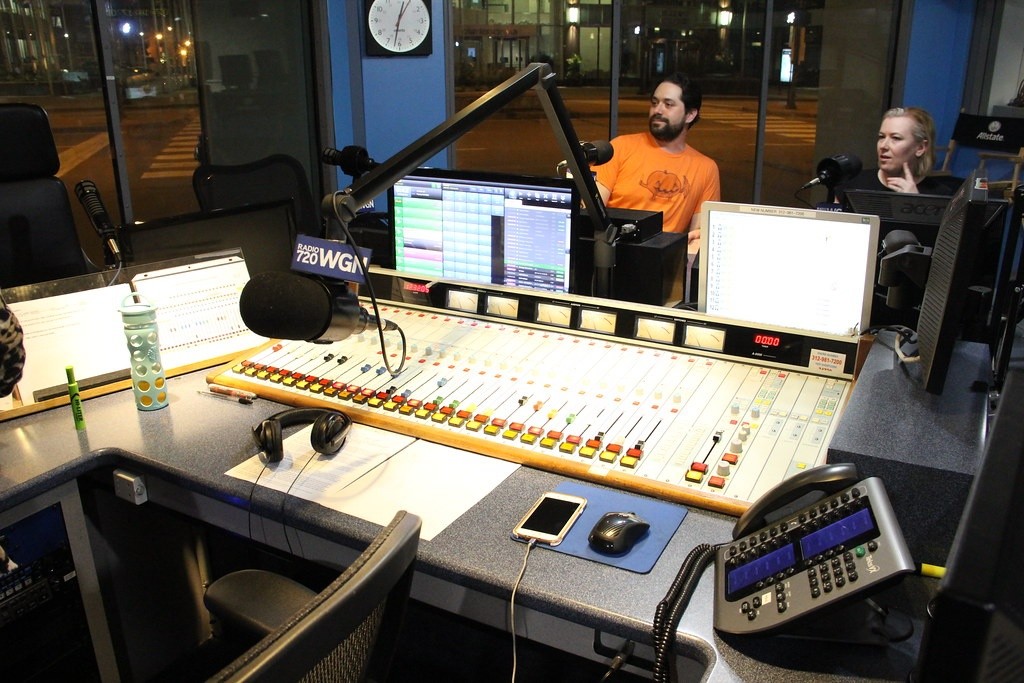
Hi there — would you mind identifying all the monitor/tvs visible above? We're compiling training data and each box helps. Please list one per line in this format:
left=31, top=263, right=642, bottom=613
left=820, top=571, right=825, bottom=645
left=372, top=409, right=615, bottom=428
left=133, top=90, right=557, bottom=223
left=386, top=168, right=582, bottom=298
left=836, top=188, right=1008, bottom=344
left=880, top=170, right=989, bottom=396
left=697, top=201, right=879, bottom=337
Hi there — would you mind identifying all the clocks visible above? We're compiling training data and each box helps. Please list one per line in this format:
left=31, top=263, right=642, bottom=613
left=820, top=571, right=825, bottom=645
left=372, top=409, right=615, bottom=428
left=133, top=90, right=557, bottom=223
left=365, top=0, right=432, bottom=57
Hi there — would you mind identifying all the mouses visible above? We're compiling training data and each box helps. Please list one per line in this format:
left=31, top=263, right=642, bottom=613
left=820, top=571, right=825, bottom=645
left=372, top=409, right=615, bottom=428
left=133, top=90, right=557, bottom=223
left=587, top=511, right=648, bottom=557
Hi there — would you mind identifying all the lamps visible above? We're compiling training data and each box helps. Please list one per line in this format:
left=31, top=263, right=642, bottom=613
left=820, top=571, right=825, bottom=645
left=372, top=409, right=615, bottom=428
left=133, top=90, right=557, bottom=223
left=566, top=4, right=579, bottom=27
left=720, top=9, right=732, bottom=26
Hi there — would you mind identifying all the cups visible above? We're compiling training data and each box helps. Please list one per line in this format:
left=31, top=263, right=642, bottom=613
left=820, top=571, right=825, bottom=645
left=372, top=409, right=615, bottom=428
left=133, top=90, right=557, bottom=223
left=119, top=303, right=169, bottom=411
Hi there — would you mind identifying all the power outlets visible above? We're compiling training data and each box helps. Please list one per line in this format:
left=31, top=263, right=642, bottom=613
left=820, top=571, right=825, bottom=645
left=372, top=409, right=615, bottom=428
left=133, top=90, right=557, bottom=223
left=113, top=469, right=148, bottom=505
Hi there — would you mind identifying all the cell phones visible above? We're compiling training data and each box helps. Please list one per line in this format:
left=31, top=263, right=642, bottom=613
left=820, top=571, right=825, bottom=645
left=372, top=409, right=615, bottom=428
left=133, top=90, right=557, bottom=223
left=511, top=492, right=588, bottom=547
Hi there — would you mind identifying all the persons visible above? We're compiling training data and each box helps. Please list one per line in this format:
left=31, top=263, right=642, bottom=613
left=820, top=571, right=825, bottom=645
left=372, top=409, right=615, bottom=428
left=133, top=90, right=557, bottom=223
left=834, top=107, right=953, bottom=213
left=580, top=75, right=721, bottom=243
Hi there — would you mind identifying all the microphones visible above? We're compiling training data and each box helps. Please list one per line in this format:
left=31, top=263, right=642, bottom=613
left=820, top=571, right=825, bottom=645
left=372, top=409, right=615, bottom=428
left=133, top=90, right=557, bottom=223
left=558, top=140, right=614, bottom=168
left=75, top=179, right=123, bottom=262
left=239, top=272, right=397, bottom=342
left=801, top=152, right=863, bottom=190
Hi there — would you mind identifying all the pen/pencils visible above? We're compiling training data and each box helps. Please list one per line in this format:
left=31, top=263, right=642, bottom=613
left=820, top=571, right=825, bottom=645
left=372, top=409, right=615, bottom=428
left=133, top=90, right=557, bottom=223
left=197, top=384, right=257, bottom=405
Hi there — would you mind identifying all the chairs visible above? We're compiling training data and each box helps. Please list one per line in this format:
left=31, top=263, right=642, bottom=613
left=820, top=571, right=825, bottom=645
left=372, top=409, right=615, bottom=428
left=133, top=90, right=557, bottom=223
left=186, top=510, right=422, bottom=683
left=191, top=153, right=322, bottom=239
left=0, top=105, right=101, bottom=289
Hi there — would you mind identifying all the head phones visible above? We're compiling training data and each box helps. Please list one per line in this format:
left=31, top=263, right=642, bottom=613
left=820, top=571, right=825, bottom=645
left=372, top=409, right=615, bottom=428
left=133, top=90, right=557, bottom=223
left=252, top=407, right=351, bottom=464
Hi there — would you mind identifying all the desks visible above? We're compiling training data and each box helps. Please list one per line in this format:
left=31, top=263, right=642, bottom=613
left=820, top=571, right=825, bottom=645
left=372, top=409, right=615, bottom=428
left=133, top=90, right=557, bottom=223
left=0, top=365, right=924, bottom=683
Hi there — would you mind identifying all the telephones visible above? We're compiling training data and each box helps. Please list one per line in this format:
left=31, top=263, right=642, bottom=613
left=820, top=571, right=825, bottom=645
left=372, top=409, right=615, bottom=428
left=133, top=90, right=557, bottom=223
left=714, top=460, right=917, bottom=639
